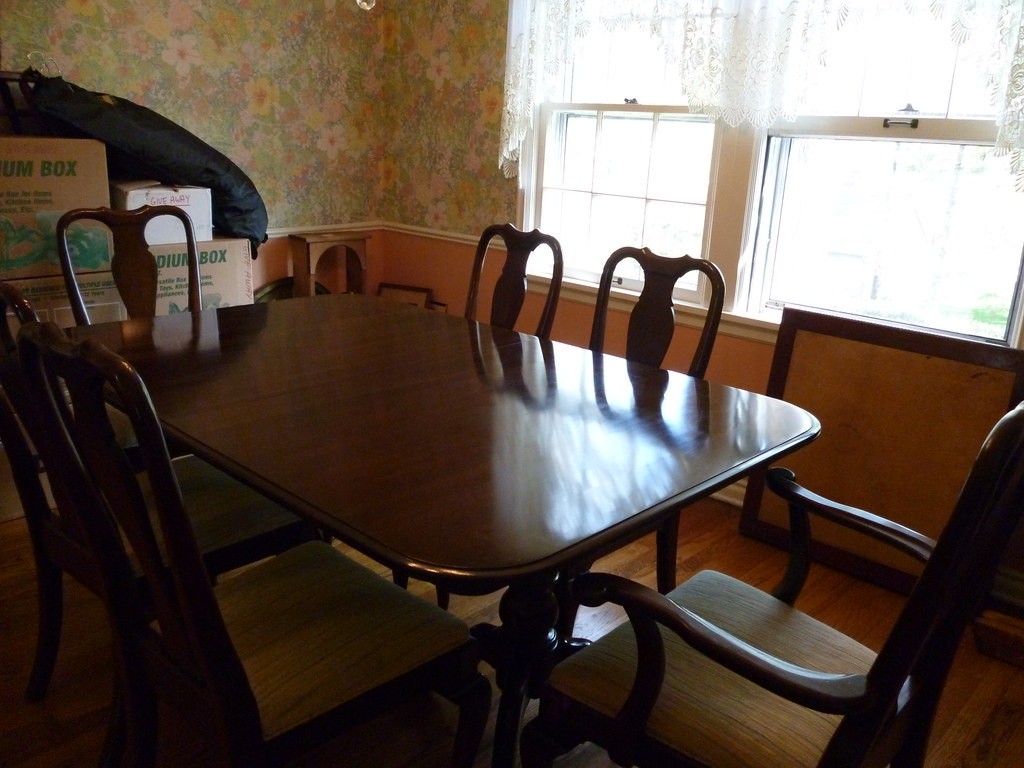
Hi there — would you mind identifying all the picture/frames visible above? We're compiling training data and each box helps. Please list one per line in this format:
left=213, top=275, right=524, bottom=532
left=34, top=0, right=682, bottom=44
left=377, top=282, right=432, bottom=310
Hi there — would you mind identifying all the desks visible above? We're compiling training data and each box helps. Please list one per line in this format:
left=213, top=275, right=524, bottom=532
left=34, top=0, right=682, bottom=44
left=288, top=232, right=373, bottom=295
left=65, top=294, right=822, bottom=768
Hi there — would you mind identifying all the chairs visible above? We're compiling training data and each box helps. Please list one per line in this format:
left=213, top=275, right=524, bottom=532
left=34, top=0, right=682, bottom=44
left=55, top=203, right=202, bottom=326
left=14, top=321, right=492, bottom=768
left=0, top=281, right=323, bottom=768
left=514, top=402, right=1024, bottom=768
left=437, top=248, right=724, bottom=612
left=464, top=224, right=562, bottom=339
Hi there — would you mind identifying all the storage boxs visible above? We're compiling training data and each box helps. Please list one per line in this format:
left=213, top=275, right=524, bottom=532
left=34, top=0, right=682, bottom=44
left=0, top=272, right=131, bottom=407
left=0, top=136, right=116, bottom=280
left=111, top=179, right=214, bottom=245
left=128, top=235, right=256, bottom=320
left=0, top=395, right=136, bottom=522
